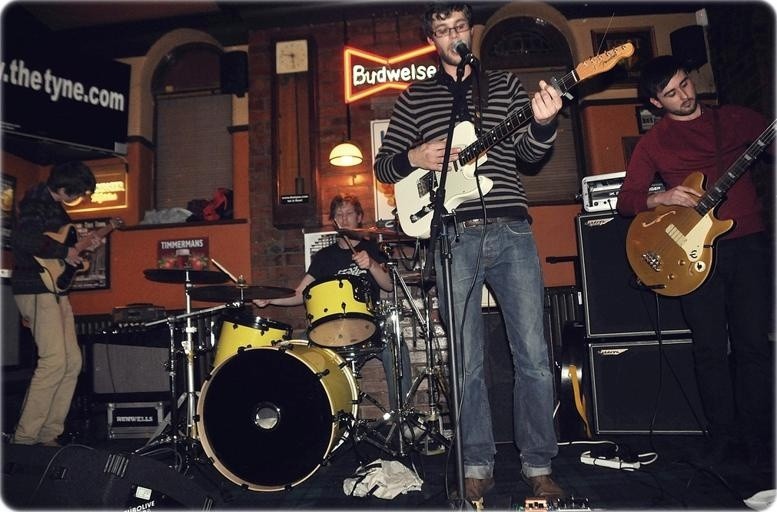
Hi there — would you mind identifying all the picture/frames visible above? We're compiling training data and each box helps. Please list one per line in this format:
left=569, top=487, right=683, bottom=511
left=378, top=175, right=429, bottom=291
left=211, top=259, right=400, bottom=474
left=635, top=105, right=656, bottom=135
left=65, top=217, right=113, bottom=288
left=371, top=120, right=394, bottom=221
left=0, top=173, right=19, bottom=250
left=590, top=24, right=657, bottom=89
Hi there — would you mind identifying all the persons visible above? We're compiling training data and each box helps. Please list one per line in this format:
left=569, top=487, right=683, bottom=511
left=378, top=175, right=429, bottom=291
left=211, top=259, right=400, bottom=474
left=251, top=192, right=427, bottom=440
left=373, top=1, right=565, bottom=501
left=9, top=160, right=103, bottom=447
left=616, top=55, right=775, bottom=485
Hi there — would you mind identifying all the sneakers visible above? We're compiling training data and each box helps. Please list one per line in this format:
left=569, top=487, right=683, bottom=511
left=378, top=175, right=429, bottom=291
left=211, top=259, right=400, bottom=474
left=450, top=471, right=498, bottom=505
left=520, top=466, right=570, bottom=501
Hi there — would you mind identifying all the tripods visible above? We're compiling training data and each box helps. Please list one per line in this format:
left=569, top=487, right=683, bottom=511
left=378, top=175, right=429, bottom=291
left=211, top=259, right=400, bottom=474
left=350, top=267, right=453, bottom=462
left=402, top=274, right=453, bottom=429
left=143, top=272, right=201, bottom=448
left=131, top=315, right=202, bottom=468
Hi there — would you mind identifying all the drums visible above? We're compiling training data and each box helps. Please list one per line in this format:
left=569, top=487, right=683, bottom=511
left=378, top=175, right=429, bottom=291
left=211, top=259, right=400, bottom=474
left=303, top=276, right=377, bottom=346
left=210, top=310, right=292, bottom=370
left=193, top=338, right=359, bottom=490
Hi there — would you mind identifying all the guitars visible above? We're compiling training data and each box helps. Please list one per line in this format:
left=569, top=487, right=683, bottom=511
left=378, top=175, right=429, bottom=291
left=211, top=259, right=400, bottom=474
left=34, top=218, right=125, bottom=294
left=625, top=119, right=776, bottom=296
left=394, top=39, right=636, bottom=240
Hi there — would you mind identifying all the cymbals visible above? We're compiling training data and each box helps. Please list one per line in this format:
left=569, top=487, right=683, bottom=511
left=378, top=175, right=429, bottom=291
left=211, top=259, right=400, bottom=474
left=339, top=226, right=412, bottom=246
left=187, top=286, right=297, bottom=302
left=141, top=268, right=231, bottom=283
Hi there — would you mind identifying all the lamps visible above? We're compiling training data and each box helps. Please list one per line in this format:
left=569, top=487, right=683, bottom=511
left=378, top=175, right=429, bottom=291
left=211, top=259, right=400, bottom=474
left=329, top=104, right=364, bottom=167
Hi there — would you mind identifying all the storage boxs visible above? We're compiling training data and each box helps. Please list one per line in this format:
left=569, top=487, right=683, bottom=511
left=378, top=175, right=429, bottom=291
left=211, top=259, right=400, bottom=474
left=107, top=401, right=164, bottom=441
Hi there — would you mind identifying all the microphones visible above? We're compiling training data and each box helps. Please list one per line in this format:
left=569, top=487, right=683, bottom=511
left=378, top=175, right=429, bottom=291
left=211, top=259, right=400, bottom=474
left=449, top=39, right=479, bottom=73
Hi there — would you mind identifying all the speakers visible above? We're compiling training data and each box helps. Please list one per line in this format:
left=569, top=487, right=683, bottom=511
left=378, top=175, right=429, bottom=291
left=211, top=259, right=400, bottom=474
left=219, top=51, right=248, bottom=97
left=670, top=27, right=709, bottom=70
left=575, top=211, right=709, bottom=440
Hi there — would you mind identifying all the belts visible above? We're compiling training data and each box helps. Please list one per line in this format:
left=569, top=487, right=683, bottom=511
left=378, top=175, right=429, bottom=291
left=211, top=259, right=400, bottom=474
left=444, top=213, right=531, bottom=229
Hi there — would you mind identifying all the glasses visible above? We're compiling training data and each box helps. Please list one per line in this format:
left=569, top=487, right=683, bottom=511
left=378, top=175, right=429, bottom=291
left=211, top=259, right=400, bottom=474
left=427, top=22, right=472, bottom=36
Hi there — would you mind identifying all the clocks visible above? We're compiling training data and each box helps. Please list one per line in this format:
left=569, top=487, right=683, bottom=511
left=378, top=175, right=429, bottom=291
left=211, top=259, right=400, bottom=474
left=273, top=38, right=310, bottom=76
